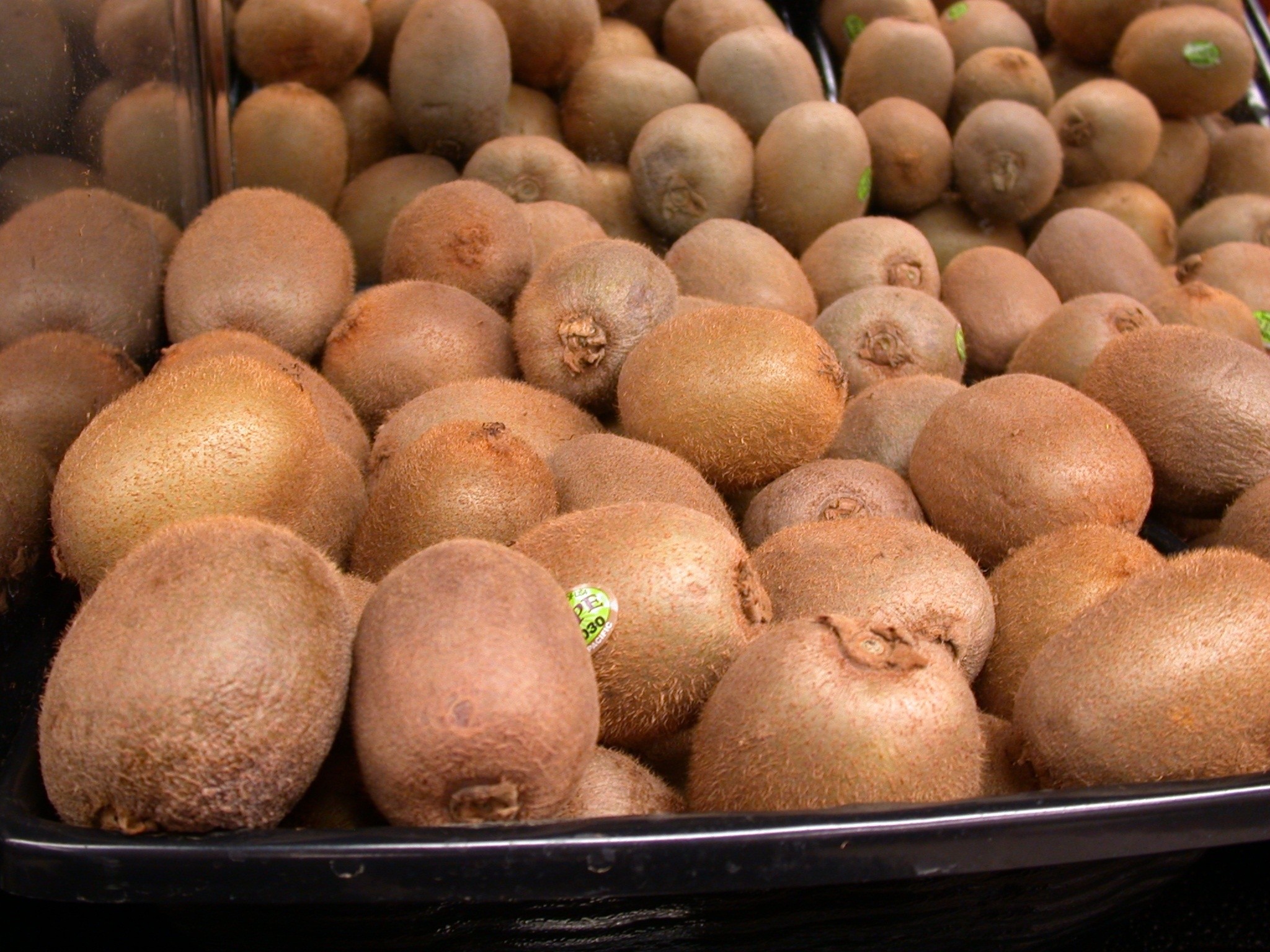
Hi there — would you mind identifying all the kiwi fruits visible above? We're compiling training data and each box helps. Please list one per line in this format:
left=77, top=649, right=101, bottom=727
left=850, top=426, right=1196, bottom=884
left=0, top=0, right=1270, bottom=821
